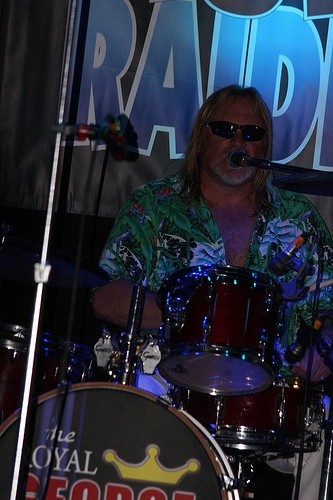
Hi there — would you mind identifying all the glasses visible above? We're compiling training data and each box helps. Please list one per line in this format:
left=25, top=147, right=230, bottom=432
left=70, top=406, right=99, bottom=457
left=206, top=121, right=267, bottom=141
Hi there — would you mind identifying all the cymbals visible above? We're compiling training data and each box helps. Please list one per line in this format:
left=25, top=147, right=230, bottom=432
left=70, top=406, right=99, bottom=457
left=0, top=234, right=107, bottom=289
left=271, top=171, right=333, bottom=197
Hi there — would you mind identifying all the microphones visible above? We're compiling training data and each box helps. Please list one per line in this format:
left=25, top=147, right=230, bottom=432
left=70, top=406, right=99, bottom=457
left=226, top=146, right=247, bottom=169
left=285, top=316, right=325, bottom=364
left=269, top=232, right=309, bottom=276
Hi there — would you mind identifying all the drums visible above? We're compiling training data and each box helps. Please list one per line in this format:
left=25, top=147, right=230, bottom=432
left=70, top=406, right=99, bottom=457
left=0, top=381, right=259, bottom=500
left=176, top=376, right=325, bottom=453
left=155, top=264, right=286, bottom=397
left=0, top=320, right=97, bottom=428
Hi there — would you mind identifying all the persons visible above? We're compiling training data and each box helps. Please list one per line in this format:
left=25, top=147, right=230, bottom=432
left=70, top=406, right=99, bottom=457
left=89, top=84, right=333, bottom=382
